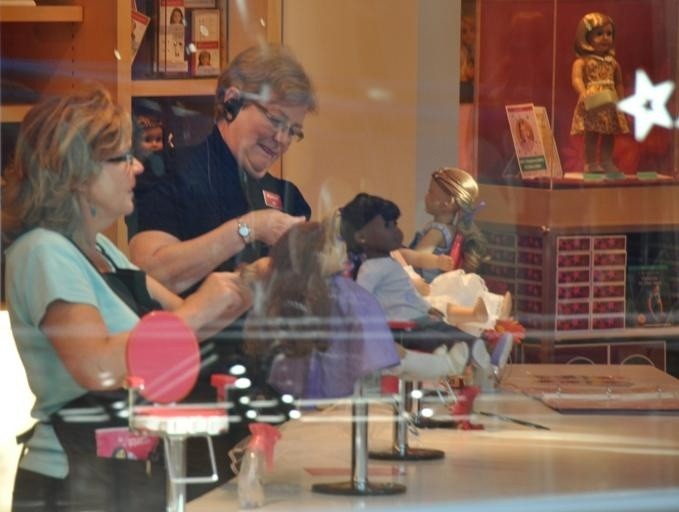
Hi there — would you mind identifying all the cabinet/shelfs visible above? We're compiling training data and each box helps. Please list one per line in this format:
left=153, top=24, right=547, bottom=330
left=0, top=1, right=131, bottom=313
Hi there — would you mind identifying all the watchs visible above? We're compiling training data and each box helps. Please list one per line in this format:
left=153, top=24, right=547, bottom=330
left=232, top=219, right=252, bottom=249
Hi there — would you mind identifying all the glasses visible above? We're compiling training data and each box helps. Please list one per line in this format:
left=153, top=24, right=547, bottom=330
left=108, top=151, right=132, bottom=164
left=254, top=100, right=303, bottom=142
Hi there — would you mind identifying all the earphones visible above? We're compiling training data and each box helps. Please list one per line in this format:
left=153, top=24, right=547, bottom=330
left=223, top=100, right=243, bottom=122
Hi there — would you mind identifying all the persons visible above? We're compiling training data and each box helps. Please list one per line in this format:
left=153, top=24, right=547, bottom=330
left=569, top=11, right=630, bottom=178
left=250, top=167, right=311, bottom=282
left=131, top=1, right=220, bottom=162
left=250, top=167, right=515, bottom=408
left=3, top=88, right=254, bottom=512
left=126, top=43, right=320, bottom=503
left=263, top=168, right=515, bottom=399
